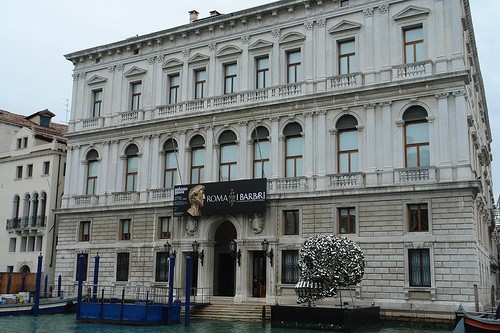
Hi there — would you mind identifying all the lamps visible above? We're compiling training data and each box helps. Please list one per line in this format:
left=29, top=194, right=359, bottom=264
left=227, top=239, right=241, bottom=267
left=260, top=238, right=274, bottom=268
left=163, top=240, right=177, bottom=259
left=191, top=240, right=204, bottom=267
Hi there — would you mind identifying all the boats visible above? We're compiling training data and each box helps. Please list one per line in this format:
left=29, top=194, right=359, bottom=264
left=1, top=295, right=89, bottom=315
left=455, top=310, right=500, bottom=333
